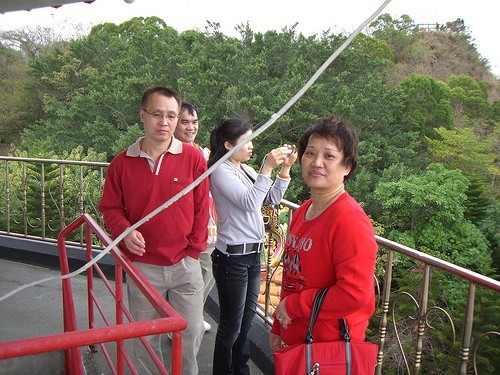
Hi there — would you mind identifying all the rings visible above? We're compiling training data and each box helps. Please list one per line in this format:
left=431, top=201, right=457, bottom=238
left=278, top=318, right=282, bottom=321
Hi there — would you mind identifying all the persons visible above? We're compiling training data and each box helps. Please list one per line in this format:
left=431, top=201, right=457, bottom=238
left=98, top=87, right=210, bottom=375
left=208, top=117, right=298, bottom=375
left=269, top=117, right=378, bottom=375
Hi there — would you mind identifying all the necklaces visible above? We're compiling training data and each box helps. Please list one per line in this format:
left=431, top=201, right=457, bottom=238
left=306, top=199, right=333, bottom=221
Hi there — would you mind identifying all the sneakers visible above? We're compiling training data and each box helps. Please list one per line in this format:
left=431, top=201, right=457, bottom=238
left=204, top=320, right=211, bottom=331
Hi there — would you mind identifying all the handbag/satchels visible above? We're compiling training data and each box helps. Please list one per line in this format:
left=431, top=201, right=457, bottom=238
left=273, top=287, right=378, bottom=375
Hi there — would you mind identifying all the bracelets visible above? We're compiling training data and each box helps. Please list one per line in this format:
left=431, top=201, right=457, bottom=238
left=278, top=172, right=290, bottom=179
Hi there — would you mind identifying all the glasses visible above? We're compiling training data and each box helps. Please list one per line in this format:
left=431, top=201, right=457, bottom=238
left=143, top=109, right=180, bottom=120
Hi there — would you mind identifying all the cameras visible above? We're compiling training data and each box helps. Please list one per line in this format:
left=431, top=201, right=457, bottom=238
left=281, top=147, right=292, bottom=156
